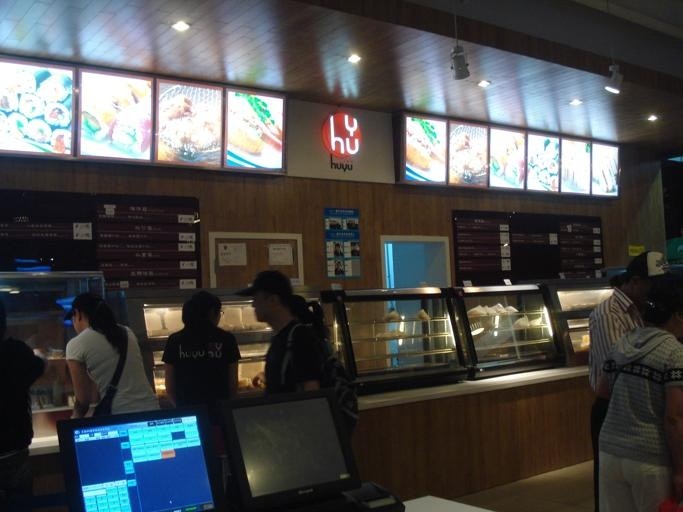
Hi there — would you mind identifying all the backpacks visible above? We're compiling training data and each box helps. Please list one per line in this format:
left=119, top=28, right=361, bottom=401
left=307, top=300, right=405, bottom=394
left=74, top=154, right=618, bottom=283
left=281, top=323, right=364, bottom=439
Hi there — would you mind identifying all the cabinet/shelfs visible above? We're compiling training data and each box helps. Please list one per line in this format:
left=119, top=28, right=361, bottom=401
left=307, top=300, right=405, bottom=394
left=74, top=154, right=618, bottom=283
left=1, top=276, right=103, bottom=412
left=340, top=286, right=614, bottom=397
left=136, top=289, right=322, bottom=409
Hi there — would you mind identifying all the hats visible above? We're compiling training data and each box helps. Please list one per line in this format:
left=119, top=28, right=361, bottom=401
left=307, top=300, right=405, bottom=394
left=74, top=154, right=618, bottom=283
left=628, top=251, right=673, bottom=278
left=64, top=293, right=104, bottom=319
left=234, top=271, right=292, bottom=295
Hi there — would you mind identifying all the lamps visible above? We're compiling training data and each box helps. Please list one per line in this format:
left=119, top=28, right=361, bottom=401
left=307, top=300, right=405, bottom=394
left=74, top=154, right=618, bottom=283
left=449, top=46, right=470, bottom=80
left=605, top=65, right=623, bottom=94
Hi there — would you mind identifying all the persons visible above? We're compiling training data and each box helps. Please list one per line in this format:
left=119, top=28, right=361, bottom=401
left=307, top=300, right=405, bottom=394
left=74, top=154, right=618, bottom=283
left=24, top=304, right=74, bottom=409
left=329, top=219, right=360, bottom=275
left=252, top=294, right=333, bottom=389
left=0, top=300, right=49, bottom=512
left=161, top=290, right=241, bottom=424
left=65, top=292, right=161, bottom=419
left=235, top=269, right=321, bottom=398
left=589, top=251, right=683, bottom=512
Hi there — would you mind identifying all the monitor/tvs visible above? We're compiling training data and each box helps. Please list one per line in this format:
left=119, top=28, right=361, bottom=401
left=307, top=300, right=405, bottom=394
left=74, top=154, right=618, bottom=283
left=56, top=404, right=226, bottom=512
left=217, top=387, right=363, bottom=512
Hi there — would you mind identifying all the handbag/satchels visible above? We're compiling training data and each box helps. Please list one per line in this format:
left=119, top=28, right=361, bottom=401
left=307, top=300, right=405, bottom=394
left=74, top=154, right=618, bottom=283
left=68, top=404, right=111, bottom=474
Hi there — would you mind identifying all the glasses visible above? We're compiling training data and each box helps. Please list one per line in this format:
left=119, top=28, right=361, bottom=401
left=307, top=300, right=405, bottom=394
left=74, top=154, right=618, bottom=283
left=216, top=310, right=223, bottom=316
